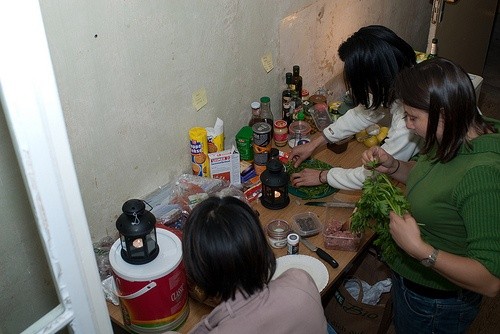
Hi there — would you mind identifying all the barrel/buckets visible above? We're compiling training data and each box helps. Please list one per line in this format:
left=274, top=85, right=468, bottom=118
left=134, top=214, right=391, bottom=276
left=109, top=228, right=191, bottom=334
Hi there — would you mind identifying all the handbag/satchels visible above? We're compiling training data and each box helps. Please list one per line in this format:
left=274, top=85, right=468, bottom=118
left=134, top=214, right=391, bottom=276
left=325, top=276, right=392, bottom=334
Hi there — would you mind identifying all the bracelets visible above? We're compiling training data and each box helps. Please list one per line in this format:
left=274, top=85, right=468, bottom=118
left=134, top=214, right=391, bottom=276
left=387, top=160, right=400, bottom=175
left=319, top=170, right=324, bottom=184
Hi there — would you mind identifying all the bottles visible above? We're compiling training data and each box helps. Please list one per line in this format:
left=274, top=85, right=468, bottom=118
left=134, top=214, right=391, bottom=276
left=282, top=72, right=293, bottom=121
left=292, top=65, right=303, bottom=98
left=427, top=38, right=438, bottom=60
left=260, top=97, right=274, bottom=135
left=287, top=233, right=299, bottom=255
left=248, top=101, right=262, bottom=127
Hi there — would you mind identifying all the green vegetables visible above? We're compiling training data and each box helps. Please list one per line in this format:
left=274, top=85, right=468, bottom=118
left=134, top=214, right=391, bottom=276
left=351, top=158, right=426, bottom=258
left=283, top=155, right=336, bottom=199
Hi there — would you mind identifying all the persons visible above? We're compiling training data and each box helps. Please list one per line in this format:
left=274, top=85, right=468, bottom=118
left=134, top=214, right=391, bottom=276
left=362, top=57, right=500, bottom=334
left=288, top=25, right=425, bottom=191
left=182, top=196, right=330, bottom=334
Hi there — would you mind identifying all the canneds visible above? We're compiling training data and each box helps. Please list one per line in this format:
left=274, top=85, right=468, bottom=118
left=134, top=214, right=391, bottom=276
left=252, top=120, right=288, bottom=166
left=301, top=89, right=343, bottom=134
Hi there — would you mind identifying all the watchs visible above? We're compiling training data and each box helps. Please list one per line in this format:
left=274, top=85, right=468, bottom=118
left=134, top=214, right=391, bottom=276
left=421, top=249, right=439, bottom=267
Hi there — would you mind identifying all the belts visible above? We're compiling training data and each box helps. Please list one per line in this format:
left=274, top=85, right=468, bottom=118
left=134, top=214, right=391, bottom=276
left=393, top=272, right=482, bottom=299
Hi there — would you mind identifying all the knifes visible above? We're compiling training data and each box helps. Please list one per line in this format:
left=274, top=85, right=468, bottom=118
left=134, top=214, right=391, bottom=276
left=298, top=236, right=339, bottom=269
left=304, top=202, right=357, bottom=208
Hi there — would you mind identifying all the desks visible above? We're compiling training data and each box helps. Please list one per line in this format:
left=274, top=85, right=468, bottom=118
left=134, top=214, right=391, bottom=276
left=106, top=72, right=405, bottom=334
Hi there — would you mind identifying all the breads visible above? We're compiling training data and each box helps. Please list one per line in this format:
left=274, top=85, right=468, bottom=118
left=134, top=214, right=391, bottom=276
left=168, top=183, right=206, bottom=216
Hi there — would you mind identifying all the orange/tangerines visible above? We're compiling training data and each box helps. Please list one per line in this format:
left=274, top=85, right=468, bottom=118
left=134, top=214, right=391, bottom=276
left=356, top=126, right=389, bottom=147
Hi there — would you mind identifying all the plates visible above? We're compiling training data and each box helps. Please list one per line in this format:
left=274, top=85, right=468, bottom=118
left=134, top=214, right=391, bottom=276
left=269, top=254, right=330, bottom=295
